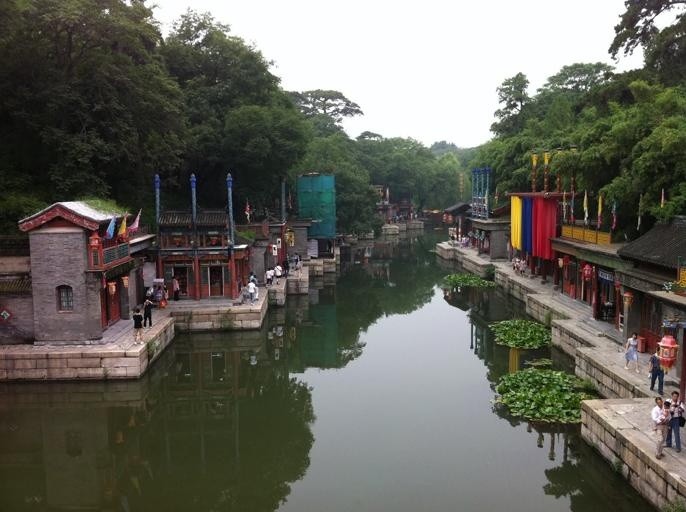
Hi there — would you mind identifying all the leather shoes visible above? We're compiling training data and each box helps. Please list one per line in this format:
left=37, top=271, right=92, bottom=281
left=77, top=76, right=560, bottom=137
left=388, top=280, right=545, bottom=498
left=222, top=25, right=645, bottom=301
left=624, top=365, right=629, bottom=370
left=658, top=389, right=664, bottom=395
left=662, top=442, right=672, bottom=447
left=675, top=447, right=682, bottom=453
left=635, top=369, right=640, bottom=374
left=650, top=387, right=654, bottom=391
left=655, top=451, right=665, bottom=460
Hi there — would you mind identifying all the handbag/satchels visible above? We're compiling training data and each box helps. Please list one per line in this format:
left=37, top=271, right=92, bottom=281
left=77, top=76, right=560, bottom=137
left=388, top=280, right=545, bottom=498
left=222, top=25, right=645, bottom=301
left=679, top=416, right=686, bottom=427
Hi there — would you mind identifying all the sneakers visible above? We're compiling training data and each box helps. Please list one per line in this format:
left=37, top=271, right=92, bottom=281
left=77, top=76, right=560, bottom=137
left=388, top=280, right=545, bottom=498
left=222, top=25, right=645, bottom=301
left=149, top=326, right=153, bottom=329
left=143, top=327, right=146, bottom=329
left=133, top=342, right=137, bottom=345
left=140, top=341, right=144, bottom=345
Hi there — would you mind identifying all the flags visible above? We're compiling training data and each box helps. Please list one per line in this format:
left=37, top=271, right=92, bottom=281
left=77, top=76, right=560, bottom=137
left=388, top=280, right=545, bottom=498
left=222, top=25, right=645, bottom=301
left=562, top=189, right=618, bottom=234
left=102, top=209, right=142, bottom=243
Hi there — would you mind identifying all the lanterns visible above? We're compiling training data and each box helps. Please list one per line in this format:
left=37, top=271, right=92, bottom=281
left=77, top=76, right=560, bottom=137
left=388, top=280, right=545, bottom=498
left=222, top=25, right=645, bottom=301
left=584, top=265, right=593, bottom=280
left=90, top=231, right=100, bottom=250
left=658, top=334, right=680, bottom=369
left=121, top=276, right=130, bottom=289
left=623, top=290, right=633, bottom=306
left=107, top=281, right=117, bottom=296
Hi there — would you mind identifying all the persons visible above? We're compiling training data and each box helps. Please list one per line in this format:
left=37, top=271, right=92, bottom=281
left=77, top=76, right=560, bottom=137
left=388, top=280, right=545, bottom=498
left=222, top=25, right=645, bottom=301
left=511, top=255, right=528, bottom=275
left=241, top=271, right=259, bottom=304
left=648, top=346, right=665, bottom=395
left=624, top=332, right=640, bottom=374
left=146, top=284, right=168, bottom=310
left=143, top=299, right=154, bottom=329
left=132, top=308, right=145, bottom=345
left=289, top=252, right=304, bottom=277
left=652, top=391, right=686, bottom=460
left=172, top=276, right=181, bottom=301
left=461, top=234, right=470, bottom=248
left=282, top=258, right=290, bottom=278
left=266, top=263, right=282, bottom=285
